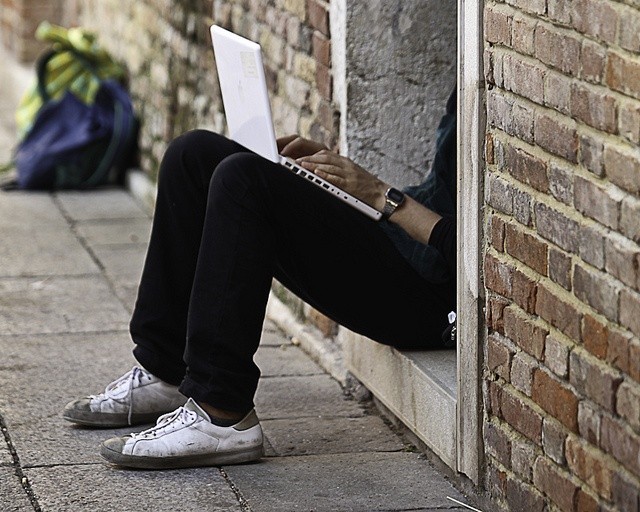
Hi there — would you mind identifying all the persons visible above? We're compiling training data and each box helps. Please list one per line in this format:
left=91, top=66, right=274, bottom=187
left=61, top=80, right=456, bottom=471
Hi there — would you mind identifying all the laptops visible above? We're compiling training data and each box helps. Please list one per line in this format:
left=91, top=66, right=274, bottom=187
left=210, top=23, right=383, bottom=222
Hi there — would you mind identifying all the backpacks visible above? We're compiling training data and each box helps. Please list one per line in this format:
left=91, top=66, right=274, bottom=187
left=13, top=41, right=138, bottom=191
left=1, top=20, right=131, bottom=173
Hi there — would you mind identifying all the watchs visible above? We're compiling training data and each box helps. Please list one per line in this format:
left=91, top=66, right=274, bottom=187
left=379, top=187, right=407, bottom=220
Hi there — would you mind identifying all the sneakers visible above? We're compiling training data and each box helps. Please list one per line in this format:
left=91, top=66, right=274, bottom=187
left=63, top=365, right=188, bottom=428
left=101, top=397, right=264, bottom=471
